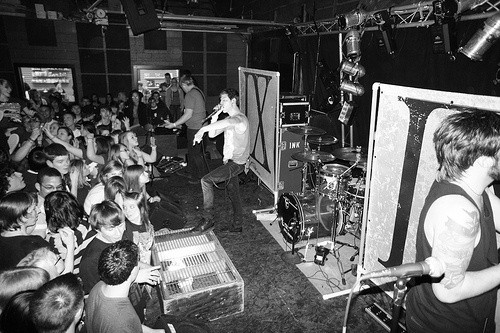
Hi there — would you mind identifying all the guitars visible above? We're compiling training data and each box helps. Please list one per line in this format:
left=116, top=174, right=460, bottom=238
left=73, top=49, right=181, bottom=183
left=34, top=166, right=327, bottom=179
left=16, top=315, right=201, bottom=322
left=154, top=120, right=187, bottom=137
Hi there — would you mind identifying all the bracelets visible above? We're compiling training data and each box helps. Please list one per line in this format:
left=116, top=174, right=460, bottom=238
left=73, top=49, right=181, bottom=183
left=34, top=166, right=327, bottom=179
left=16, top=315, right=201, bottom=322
left=147, top=197, right=151, bottom=201
left=29, top=137, right=35, bottom=142
left=50, top=136, right=55, bottom=139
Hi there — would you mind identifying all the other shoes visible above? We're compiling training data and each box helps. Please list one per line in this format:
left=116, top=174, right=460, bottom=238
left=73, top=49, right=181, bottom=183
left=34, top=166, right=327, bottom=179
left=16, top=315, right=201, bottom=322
left=176, top=170, right=192, bottom=178
left=189, top=178, right=201, bottom=184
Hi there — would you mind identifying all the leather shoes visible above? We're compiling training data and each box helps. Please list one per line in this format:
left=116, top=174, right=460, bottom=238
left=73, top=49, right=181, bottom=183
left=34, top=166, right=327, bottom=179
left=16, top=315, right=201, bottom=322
left=220, top=225, right=242, bottom=235
left=189, top=218, right=214, bottom=236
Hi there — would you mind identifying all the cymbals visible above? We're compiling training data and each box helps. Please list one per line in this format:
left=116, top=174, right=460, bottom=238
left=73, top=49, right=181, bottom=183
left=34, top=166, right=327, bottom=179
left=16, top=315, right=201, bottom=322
left=301, top=134, right=338, bottom=145
left=333, top=146, right=368, bottom=162
left=291, top=150, right=335, bottom=163
left=287, top=125, right=328, bottom=135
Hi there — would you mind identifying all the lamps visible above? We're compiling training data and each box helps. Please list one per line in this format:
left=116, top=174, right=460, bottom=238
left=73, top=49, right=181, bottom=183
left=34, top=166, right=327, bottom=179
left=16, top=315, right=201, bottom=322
left=338, top=59, right=366, bottom=83
left=433, top=0, right=485, bottom=26
left=343, top=29, right=362, bottom=59
left=460, top=12, right=500, bottom=61
left=441, top=21, right=459, bottom=61
left=340, top=79, right=366, bottom=96
left=337, top=8, right=367, bottom=32
left=373, top=10, right=397, bottom=55
left=338, top=100, right=359, bottom=126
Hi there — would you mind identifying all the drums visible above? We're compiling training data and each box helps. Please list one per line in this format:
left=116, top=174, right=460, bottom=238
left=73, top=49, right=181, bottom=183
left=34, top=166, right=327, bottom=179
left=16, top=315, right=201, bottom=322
left=315, top=163, right=352, bottom=201
left=277, top=189, right=343, bottom=244
left=346, top=162, right=367, bottom=198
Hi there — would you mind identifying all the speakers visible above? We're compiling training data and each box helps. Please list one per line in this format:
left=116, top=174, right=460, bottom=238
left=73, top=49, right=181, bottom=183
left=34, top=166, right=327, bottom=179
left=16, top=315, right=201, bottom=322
left=118, top=0, right=161, bottom=37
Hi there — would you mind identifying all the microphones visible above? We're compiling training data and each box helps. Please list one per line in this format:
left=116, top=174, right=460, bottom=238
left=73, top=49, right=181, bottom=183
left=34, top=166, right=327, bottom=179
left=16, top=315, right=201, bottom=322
left=201, top=106, right=223, bottom=122
left=361, top=256, right=445, bottom=281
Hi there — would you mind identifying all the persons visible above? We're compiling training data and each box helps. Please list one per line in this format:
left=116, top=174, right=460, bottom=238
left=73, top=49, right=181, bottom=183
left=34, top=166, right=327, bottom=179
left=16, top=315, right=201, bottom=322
left=162, top=74, right=207, bottom=184
left=404, top=109, right=500, bottom=333
left=191, top=88, right=252, bottom=233
left=0, top=78, right=165, bottom=333
left=160, top=72, right=185, bottom=123
